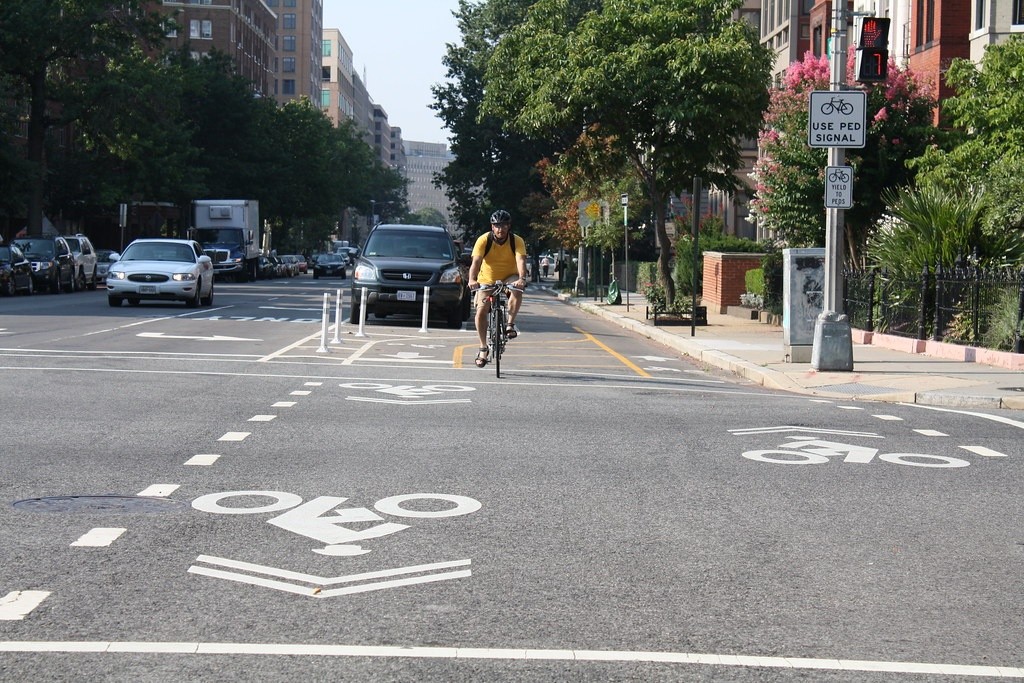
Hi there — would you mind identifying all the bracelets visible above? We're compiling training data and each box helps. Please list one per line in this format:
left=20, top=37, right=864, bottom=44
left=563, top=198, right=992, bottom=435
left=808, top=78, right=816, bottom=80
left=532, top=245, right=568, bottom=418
left=519, top=277, right=526, bottom=281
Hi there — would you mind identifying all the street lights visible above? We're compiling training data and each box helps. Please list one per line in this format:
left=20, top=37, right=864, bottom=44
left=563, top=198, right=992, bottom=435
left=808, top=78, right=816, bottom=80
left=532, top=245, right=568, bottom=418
left=368, top=200, right=394, bottom=224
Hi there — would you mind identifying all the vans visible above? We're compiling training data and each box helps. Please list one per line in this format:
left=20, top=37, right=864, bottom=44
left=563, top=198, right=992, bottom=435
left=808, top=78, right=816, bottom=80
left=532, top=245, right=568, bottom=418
left=525, top=255, right=559, bottom=277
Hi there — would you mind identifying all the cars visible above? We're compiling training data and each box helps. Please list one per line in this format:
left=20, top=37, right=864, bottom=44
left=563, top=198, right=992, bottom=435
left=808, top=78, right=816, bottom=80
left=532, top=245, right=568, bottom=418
left=106, top=239, right=215, bottom=308
left=0, top=242, right=34, bottom=297
left=337, top=247, right=358, bottom=265
left=308, top=251, right=351, bottom=279
left=260, top=254, right=308, bottom=280
left=95, top=249, right=120, bottom=283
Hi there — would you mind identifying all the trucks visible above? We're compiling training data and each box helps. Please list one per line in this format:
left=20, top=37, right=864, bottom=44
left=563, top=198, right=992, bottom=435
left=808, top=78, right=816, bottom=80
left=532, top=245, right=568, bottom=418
left=190, top=199, right=260, bottom=283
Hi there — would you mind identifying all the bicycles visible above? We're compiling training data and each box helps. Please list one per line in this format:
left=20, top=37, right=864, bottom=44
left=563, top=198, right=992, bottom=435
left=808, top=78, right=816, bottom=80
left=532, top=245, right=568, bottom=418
left=471, top=280, right=524, bottom=379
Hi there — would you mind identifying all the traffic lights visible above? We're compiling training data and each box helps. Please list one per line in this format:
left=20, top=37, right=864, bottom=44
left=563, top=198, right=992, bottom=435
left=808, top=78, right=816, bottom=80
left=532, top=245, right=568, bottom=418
left=853, top=17, right=891, bottom=82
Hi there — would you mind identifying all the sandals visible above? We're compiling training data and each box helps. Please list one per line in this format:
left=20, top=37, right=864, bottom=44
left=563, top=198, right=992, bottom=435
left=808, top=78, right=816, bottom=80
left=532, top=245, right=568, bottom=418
left=506, top=322, right=517, bottom=339
left=475, top=345, right=489, bottom=367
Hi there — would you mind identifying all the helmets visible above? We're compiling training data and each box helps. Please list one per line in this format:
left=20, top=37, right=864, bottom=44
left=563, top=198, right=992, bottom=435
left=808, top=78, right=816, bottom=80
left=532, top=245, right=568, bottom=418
left=490, top=209, right=511, bottom=224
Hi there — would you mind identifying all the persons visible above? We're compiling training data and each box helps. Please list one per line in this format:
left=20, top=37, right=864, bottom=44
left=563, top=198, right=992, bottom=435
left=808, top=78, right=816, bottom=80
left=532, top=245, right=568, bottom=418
left=541, top=255, right=549, bottom=278
left=271, top=247, right=277, bottom=258
left=468, top=210, right=527, bottom=368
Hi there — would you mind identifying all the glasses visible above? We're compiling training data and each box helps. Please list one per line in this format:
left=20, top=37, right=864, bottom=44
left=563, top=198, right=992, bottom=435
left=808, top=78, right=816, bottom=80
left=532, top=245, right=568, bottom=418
left=494, top=223, right=508, bottom=228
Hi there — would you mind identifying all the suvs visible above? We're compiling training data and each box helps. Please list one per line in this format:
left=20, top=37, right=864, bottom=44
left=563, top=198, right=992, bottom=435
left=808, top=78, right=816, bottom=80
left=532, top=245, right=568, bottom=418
left=348, top=222, right=464, bottom=329
left=10, top=233, right=97, bottom=295
